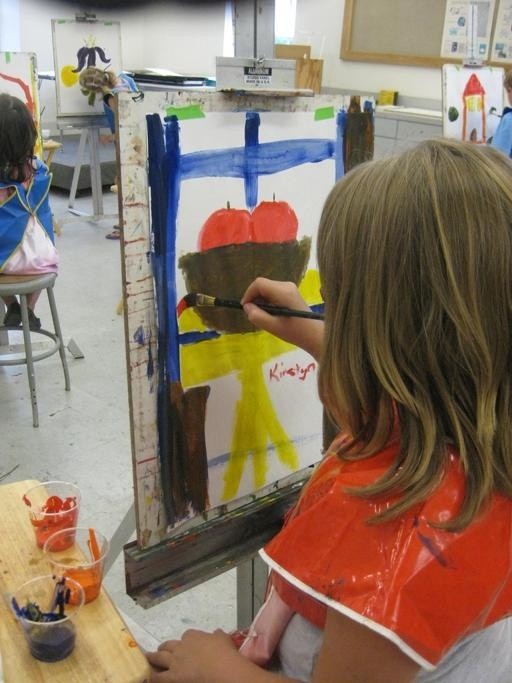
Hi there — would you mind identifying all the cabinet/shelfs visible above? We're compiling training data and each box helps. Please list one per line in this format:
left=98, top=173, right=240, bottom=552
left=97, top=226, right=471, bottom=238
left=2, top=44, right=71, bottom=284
left=374, top=118, right=440, bottom=162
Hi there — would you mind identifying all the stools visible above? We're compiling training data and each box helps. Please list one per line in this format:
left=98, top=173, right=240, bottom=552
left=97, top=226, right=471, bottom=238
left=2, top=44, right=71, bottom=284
left=1, top=273, right=71, bottom=426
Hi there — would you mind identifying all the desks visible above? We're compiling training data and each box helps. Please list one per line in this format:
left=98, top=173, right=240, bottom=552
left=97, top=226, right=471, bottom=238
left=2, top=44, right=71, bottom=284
left=2, top=478, right=158, bottom=682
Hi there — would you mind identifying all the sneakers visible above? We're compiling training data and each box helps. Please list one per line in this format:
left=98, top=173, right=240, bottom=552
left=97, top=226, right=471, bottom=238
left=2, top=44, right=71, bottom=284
left=4, top=300, right=22, bottom=327
left=28, top=307, right=41, bottom=330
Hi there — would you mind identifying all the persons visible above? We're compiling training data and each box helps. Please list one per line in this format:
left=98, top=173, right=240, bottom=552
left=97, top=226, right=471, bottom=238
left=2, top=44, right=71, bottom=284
left=138, top=136, right=511, bottom=682
left=0, top=91, right=53, bottom=330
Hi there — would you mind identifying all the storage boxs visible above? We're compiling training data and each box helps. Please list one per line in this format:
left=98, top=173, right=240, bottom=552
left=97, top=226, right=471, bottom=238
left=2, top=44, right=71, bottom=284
left=215, top=55, right=323, bottom=94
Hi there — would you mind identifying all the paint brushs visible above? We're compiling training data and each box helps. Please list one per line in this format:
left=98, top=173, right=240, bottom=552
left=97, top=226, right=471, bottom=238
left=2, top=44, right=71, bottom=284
left=489, top=109, right=501, bottom=117
left=184, top=293, right=325, bottom=320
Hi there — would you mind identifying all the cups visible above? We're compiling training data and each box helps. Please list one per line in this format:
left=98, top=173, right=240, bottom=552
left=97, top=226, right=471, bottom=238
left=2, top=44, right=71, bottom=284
left=42, top=527, right=110, bottom=607
left=20, top=480, right=82, bottom=554
left=9, top=576, right=85, bottom=664
left=41, top=129, right=50, bottom=141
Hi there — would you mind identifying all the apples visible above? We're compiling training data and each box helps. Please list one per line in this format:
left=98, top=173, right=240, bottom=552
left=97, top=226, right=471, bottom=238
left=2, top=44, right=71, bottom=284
left=252, top=193, right=299, bottom=243
left=197, top=201, right=252, bottom=253
